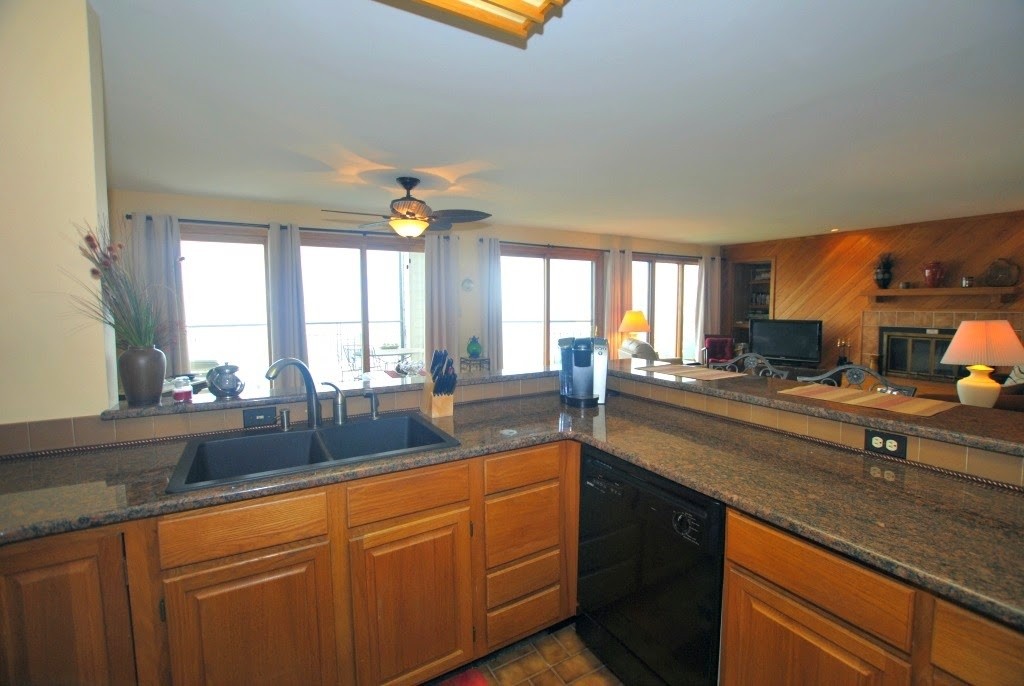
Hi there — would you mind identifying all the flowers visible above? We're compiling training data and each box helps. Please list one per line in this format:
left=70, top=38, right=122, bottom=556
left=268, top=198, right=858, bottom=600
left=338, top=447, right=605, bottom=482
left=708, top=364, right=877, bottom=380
left=36, top=212, right=185, bottom=346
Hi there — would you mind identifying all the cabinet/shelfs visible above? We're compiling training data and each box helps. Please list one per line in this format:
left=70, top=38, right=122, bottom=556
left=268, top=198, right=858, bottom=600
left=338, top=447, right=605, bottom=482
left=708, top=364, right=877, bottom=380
left=0, top=428, right=1024, bottom=686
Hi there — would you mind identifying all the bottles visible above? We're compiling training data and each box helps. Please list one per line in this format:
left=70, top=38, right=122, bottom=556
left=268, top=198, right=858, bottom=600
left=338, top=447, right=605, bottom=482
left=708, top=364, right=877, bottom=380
left=923, top=262, right=944, bottom=288
left=869, top=354, right=877, bottom=374
left=751, top=293, right=769, bottom=305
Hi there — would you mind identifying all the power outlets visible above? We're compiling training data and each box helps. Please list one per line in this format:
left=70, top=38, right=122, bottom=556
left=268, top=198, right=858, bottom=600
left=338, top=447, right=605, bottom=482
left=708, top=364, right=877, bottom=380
left=865, top=428, right=907, bottom=460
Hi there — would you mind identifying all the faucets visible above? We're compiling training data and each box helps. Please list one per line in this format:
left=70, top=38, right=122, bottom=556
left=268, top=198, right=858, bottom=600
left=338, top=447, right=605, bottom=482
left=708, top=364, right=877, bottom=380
left=265, top=358, right=346, bottom=427
left=274, top=408, right=291, bottom=431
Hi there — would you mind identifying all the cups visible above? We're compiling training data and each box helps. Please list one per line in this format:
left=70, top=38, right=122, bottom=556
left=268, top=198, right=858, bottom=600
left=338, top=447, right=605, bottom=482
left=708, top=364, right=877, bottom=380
left=961, top=276, right=974, bottom=288
left=172, top=377, right=193, bottom=403
left=900, top=282, right=909, bottom=289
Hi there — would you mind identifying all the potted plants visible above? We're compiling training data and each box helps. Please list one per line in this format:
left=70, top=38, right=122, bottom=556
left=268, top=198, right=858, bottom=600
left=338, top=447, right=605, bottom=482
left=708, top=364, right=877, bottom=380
left=872, top=252, right=899, bottom=289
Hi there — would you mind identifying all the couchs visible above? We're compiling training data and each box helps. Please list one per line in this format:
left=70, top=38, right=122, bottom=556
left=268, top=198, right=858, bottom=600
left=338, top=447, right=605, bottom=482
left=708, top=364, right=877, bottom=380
left=617, top=339, right=682, bottom=365
left=992, top=374, right=1024, bottom=411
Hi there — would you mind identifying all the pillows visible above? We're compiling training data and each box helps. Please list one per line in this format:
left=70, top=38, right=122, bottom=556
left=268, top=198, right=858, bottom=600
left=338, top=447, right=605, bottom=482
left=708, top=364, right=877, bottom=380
left=1005, top=364, right=1024, bottom=385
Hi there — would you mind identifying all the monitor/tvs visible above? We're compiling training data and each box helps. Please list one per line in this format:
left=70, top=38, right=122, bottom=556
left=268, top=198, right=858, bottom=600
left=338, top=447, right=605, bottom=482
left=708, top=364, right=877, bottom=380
left=748, top=317, right=822, bottom=370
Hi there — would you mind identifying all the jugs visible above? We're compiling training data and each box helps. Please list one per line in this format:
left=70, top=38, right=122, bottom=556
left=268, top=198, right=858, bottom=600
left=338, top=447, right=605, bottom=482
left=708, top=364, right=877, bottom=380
left=205, top=362, right=245, bottom=401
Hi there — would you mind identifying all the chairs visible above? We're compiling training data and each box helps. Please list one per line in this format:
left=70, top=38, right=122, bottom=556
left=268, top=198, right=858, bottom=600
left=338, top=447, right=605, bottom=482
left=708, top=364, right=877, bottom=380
left=709, top=353, right=789, bottom=379
left=797, top=364, right=917, bottom=397
left=342, top=344, right=387, bottom=382
left=700, top=334, right=737, bottom=371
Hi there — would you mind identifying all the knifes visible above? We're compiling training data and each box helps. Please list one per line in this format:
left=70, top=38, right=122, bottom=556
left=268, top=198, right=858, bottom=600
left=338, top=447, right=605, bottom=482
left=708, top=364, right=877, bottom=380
left=429, top=350, right=457, bottom=396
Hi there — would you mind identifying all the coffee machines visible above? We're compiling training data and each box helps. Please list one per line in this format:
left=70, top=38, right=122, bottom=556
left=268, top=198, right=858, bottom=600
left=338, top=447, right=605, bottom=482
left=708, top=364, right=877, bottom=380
left=557, top=338, right=609, bottom=406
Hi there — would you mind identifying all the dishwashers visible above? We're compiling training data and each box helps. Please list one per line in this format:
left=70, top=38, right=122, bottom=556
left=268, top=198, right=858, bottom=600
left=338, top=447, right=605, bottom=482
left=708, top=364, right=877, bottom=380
left=575, top=443, right=726, bottom=686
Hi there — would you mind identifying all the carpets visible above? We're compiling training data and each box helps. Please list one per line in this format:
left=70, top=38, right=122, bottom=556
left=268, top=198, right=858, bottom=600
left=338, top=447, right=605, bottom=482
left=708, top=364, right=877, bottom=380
left=384, top=370, right=427, bottom=378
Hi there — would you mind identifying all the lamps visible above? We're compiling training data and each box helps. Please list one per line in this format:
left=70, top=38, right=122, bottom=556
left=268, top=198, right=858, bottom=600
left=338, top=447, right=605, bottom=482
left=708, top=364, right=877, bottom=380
left=618, top=310, right=651, bottom=332
left=374, top=0, right=571, bottom=49
left=940, top=320, right=1024, bottom=408
left=388, top=199, right=432, bottom=240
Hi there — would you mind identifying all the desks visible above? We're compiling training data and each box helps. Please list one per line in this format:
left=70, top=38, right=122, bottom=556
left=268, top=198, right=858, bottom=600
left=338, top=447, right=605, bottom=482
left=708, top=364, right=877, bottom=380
left=354, top=350, right=423, bottom=363
left=460, top=357, right=490, bottom=373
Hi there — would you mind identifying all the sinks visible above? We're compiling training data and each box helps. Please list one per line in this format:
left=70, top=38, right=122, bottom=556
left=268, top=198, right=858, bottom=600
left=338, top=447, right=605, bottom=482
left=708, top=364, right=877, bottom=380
left=165, top=425, right=331, bottom=493
left=317, top=411, right=461, bottom=465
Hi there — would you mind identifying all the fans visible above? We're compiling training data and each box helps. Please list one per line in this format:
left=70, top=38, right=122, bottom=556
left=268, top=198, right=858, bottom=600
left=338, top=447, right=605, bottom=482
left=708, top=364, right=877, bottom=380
left=321, top=177, right=493, bottom=231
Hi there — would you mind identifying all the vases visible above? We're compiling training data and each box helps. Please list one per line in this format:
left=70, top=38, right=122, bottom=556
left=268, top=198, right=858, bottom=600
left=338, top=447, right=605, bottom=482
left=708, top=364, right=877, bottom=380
left=119, top=345, right=167, bottom=407
left=924, top=263, right=942, bottom=288
